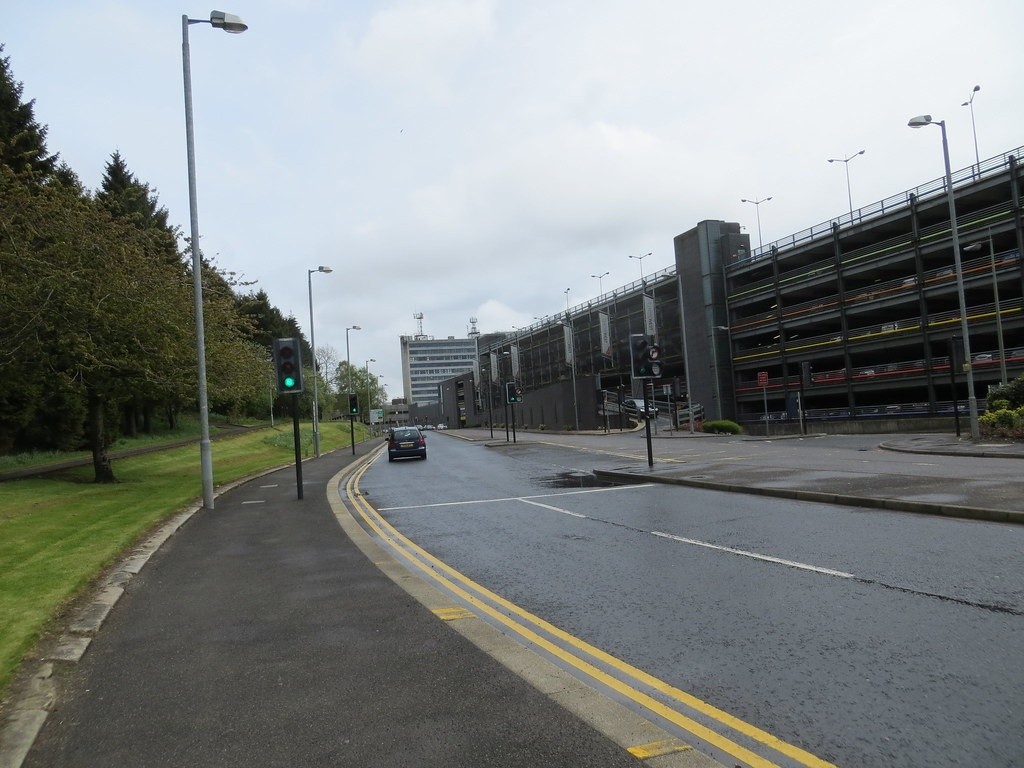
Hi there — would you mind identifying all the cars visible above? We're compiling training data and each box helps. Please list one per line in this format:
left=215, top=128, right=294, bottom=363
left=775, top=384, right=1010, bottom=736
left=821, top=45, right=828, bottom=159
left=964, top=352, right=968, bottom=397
left=416, top=424, right=447, bottom=430
left=621, top=399, right=659, bottom=419
left=386, top=427, right=428, bottom=461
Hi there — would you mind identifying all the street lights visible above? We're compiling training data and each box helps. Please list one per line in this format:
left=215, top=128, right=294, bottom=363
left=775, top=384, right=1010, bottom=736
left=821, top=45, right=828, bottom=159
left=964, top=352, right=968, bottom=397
left=628, top=252, right=652, bottom=280
left=307, top=265, right=335, bottom=455
left=907, top=115, right=985, bottom=441
left=376, top=374, right=384, bottom=409
left=182, top=11, right=248, bottom=512
left=366, top=358, right=377, bottom=439
left=344, top=326, right=362, bottom=393
left=381, top=384, right=389, bottom=425
left=826, top=149, right=868, bottom=224
left=742, top=195, right=773, bottom=256
left=591, top=272, right=610, bottom=299
left=564, top=287, right=571, bottom=309
left=961, top=85, right=985, bottom=179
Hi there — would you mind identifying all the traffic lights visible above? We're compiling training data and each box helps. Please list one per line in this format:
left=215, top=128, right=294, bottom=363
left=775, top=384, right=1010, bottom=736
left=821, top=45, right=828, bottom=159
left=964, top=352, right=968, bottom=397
left=508, top=382, right=516, bottom=403
left=630, top=333, right=650, bottom=379
left=515, top=387, right=524, bottom=403
left=273, top=337, right=305, bottom=396
left=647, top=343, right=664, bottom=381
left=348, top=393, right=360, bottom=415
